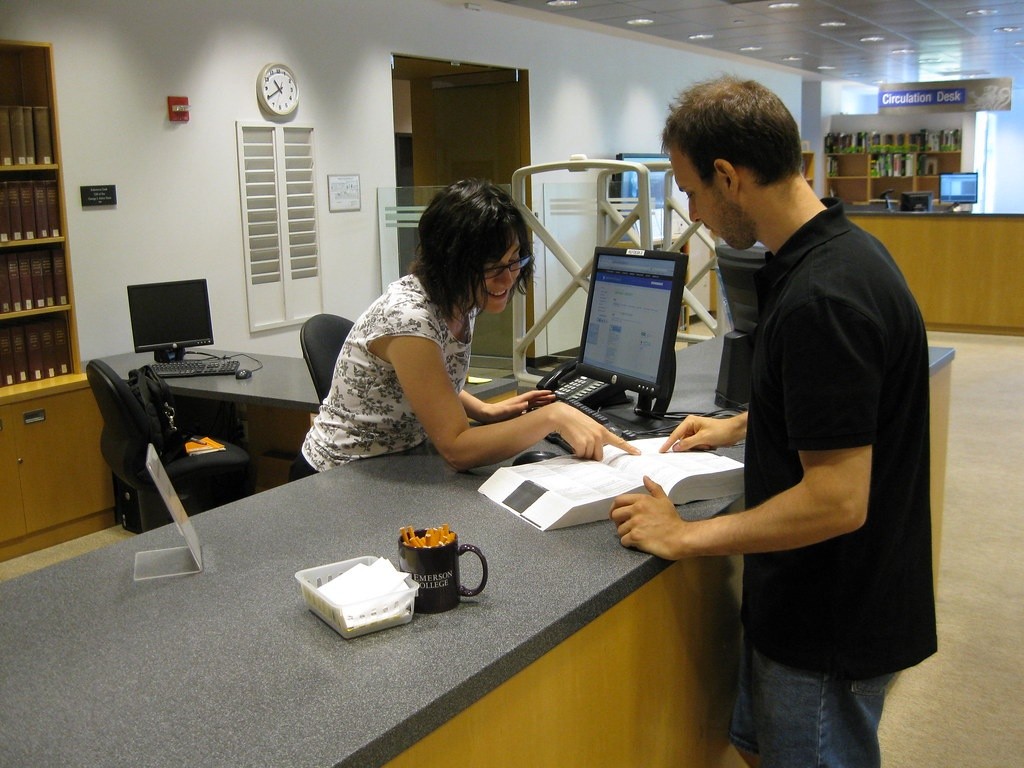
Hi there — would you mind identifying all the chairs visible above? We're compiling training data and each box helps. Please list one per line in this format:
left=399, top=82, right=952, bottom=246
left=85, top=358, right=253, bottom=512
left=300, top=313, right=355, bottom=405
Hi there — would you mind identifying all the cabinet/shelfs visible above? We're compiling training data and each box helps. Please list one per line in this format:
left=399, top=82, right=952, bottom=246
left=0, top=387, right=116, bottom=562
left=709, top=139, right=816, bottom=319
left=0, top=38, right=83, bottom=375
left=823, top=129, right=961, bottom=205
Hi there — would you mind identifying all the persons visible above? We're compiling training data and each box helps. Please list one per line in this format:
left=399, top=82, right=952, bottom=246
left=284, top=178, right=640, bottom=485
left=610, top=73, right=940, bottom=768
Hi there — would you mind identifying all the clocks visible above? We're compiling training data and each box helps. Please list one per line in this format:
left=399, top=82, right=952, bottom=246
left=255, top=62, right=300, bottom=116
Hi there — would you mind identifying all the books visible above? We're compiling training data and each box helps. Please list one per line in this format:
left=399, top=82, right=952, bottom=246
left=0, top=106, right=75, bottom=388
left=922, top=128, right=959, bottom=153
left=184, top=437, right=226, bottom=457
left=479, top=434, right=745, bottom=533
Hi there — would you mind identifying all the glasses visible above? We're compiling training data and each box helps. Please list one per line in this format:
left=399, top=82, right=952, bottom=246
left=482, top=252, right=533, bottom=279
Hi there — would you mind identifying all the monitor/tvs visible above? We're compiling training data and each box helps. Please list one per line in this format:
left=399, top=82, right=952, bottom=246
left=127, top=278, right=214, bottom=364
left=901, top=191, right=933, bottom=212
left=575, top=246, right=689, bottom=433
left=607, top=152, right=674, bottom=209
left=939, top=172, right=978, bottom=205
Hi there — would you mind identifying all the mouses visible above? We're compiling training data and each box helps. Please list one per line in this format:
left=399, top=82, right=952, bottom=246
left=236, top=369, right=252, bottom=379
left=512, top=451, right=559, bottom=466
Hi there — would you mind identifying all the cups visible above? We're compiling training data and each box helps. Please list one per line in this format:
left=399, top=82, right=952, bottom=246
left=398, top=528, right=488, bottom=614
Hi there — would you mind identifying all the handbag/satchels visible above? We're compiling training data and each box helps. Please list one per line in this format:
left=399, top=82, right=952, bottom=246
left=126, top=365, right=188, bottom=465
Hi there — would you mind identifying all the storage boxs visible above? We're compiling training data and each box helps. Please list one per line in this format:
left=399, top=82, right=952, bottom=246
left=294, top=555, right=420, bottom=639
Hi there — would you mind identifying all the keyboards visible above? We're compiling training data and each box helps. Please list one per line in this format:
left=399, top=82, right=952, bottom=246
left=151, top=360, right=240, bottom=377
left=525, top=394, right=623, bottom=452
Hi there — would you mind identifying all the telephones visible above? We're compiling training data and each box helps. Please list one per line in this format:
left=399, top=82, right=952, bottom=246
left=534, top=358, right=619, bottom=406
